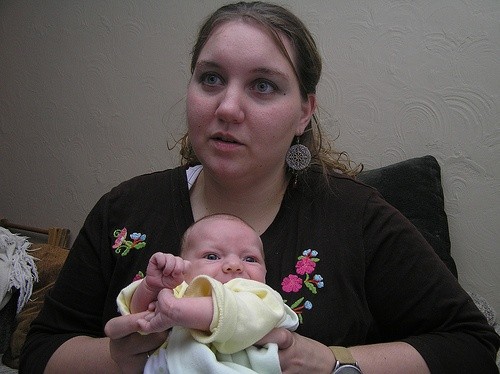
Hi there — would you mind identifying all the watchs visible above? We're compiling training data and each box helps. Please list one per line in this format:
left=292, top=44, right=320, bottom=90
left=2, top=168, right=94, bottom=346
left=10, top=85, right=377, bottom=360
left=329, top=346, right=363, bottom=374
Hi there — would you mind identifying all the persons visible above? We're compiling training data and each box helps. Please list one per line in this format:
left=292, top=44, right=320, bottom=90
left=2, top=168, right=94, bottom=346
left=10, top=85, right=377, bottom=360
left=17, top=2, right=500, bottom=374
left=116, top=212, right=299, bottom=374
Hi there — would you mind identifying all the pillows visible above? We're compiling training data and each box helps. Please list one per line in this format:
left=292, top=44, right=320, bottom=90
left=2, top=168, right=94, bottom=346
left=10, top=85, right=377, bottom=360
left=350, top=154, right=457, bottom=278
